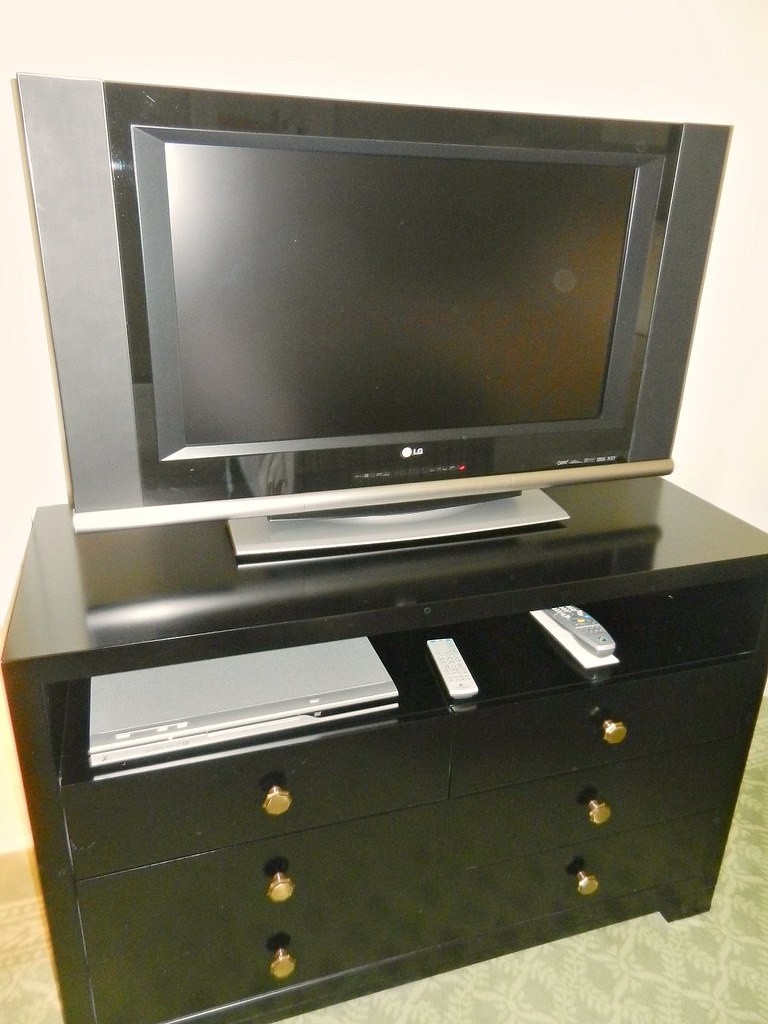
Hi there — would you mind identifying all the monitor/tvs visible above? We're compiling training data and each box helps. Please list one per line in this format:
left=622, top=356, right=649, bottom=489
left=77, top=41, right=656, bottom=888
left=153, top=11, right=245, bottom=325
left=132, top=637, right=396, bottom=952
left=14, top=75, right=735, bottom=568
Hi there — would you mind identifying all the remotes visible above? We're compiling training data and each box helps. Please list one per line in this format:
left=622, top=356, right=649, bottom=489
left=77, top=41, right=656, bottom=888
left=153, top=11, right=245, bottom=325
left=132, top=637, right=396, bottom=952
left=427, top=638, right=479, bottom=699
left=543, top=605, right=616, bottom=659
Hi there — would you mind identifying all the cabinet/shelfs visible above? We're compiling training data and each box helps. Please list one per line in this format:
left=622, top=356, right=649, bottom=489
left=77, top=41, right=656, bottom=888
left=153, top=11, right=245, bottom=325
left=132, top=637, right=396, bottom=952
left=0, top=476, right=768, bottom=1024
